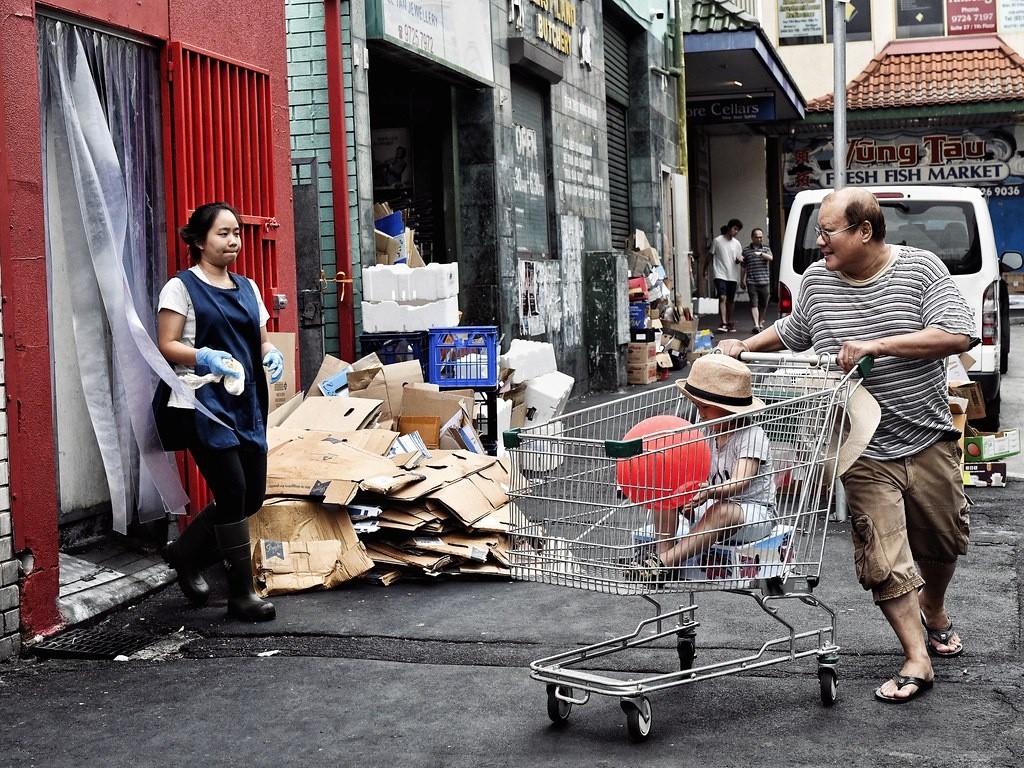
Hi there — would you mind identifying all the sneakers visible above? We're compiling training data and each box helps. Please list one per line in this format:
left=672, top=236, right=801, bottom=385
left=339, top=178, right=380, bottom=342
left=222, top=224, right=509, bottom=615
left=728, top=323, right=736, bottom=332
left=717, top=323, right=728, bottom=332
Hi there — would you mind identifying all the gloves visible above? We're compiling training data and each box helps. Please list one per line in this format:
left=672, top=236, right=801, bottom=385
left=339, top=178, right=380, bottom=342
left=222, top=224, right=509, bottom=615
left=196, top=347, right=240, bottom=380
left=262, top=349, right=284, bottom=385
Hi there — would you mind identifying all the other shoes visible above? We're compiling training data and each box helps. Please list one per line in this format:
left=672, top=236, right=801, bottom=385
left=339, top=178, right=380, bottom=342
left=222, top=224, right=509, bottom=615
left=752, top=327, right=759, bottom=334
left=760, top=325, right=764, bottom=332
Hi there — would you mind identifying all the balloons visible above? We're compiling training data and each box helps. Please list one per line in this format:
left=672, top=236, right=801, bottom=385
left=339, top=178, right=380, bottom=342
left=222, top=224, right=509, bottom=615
left=619, top=415, right=711, bottom=509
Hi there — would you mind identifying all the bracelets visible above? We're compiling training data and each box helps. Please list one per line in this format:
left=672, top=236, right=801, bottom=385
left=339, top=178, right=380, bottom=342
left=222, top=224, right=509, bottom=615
left=760, top=253, right=762, bottom=256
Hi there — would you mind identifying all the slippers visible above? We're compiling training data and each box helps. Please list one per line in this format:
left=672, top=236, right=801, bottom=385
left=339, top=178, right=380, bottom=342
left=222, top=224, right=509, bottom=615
left=875, top=671, right=935, bottom=704
left=921, top=610, right=963, bottom=658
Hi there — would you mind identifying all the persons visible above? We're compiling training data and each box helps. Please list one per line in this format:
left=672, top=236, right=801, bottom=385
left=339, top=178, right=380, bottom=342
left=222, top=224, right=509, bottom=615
left=717, top=188, right=982, bottom=702
left=740, top=228, right=773, bottom=333
left=622, top=354, right=776, bottom=580
left=704, top=218, right=742, bottom=331
left=140, top=201, right=284, bottom=621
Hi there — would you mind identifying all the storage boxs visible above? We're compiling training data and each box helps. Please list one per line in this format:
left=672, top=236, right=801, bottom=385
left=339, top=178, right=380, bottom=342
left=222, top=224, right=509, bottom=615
left=625, top=226, right=721, bottom=384
left=244, top=199, right=575, bottom=599
left=944, top=352, right=1021, bottom=486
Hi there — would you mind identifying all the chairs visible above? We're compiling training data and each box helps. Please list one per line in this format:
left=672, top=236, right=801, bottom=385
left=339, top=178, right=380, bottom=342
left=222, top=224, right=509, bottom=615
left=940, top=221, right=968, bottom=248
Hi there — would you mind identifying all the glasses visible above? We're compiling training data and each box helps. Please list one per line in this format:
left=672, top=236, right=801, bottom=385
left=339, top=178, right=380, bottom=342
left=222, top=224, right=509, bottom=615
left=814, top=223, right=859, bottom=244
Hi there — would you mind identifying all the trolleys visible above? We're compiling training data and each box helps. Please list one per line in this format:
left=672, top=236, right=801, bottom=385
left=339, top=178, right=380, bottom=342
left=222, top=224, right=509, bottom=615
left=501, top=351, right=875, bottom=742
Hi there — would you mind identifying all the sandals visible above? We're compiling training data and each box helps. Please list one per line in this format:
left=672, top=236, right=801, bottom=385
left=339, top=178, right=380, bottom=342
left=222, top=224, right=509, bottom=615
left=624, top=555, right=668, bottom=589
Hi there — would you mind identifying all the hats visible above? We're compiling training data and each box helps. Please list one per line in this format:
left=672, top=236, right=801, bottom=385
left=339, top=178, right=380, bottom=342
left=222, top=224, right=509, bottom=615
left=675, top=353, right=766, bottom=416
left=796, top=372, right=882, bottom=487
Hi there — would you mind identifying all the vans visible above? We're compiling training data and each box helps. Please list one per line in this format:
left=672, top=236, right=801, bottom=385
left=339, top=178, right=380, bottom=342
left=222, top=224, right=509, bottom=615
left=776, top=186, right=1023, bottom=404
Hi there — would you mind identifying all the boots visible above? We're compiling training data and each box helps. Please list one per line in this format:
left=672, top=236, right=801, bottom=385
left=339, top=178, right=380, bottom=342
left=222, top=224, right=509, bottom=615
left=162, top=500, right=219, bottom=602
left=220, top=518, right=276, bottom=622
